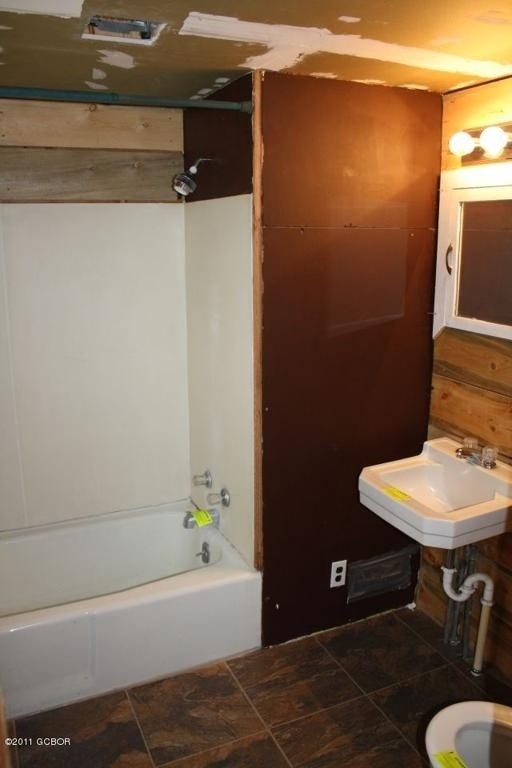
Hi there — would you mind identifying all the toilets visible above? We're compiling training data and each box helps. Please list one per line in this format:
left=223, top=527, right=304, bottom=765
left=425, top=701, right=512, bottom=768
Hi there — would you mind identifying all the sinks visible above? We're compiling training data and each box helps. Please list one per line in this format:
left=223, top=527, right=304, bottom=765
left=359, top=453, right=512, bottom=550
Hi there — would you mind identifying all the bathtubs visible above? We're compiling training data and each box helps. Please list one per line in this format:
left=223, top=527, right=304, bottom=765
left=0, top=500, right=263, bottom=721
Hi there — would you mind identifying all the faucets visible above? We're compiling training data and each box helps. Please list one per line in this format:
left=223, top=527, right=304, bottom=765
left=456, top=447, right=481, bottom=461
left=183, top=509, right=219, bottom=528
